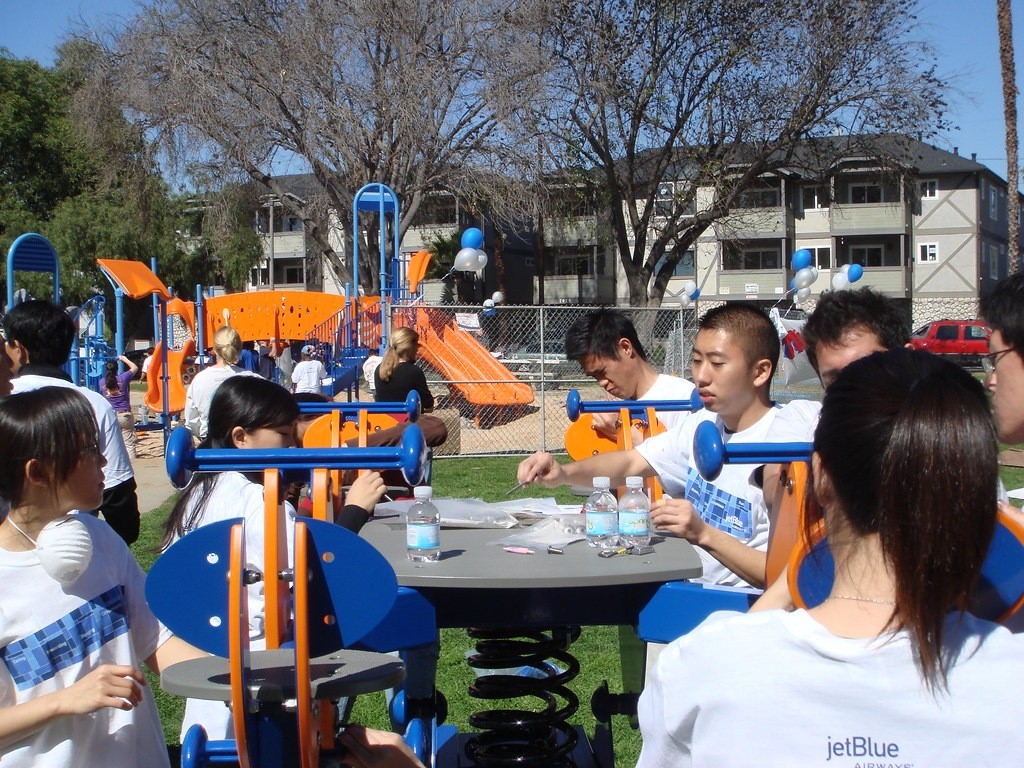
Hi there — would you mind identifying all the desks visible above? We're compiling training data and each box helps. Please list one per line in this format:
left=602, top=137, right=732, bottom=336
left=500, top=360, right=569, bottom=389
left=355, top=494, right=706, bottom=768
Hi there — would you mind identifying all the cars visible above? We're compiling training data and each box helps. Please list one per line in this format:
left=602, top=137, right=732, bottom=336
left=902, top=319, right=994, bottom=358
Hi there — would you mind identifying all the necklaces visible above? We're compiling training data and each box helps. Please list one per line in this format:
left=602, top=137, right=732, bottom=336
left=823, top=595, right=894, bottom=604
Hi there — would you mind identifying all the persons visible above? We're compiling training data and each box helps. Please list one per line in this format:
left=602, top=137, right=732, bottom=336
left=186, top=327, right=266, bottom=442
left=634, top=350, right=1023, bottom=767
left=3, top=300, right=140, bottom=544
left=565, top=309, right=698, bottom=445
left=517, top=306, right=785, bottom=590
left=764, top=289, right=914, bottom=503
left=375, top=327, right=434, bottom=410
left=0, top=335, right=428, bottom=768
left=98, top=355, right=139, bottom=458
left=985, top=270, right=1024, bottom=442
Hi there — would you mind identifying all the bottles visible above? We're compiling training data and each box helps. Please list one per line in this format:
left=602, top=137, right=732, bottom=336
left=586, top=477, right=618, bottom=547
left=618, top=476, right=651, bottom=548
left=406, top=486, right=441, bottom=562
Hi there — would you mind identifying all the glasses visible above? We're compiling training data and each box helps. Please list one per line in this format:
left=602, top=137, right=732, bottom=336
left=977, top=347, right=1020, bottom=377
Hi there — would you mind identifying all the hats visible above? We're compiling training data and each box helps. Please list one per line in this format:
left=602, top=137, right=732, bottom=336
left=301, top=345, right=316, bottom=354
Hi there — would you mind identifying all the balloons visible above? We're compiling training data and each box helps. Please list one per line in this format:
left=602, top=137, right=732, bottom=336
left=483, top=291, right=502, bottom=316
left=681, top=281, right=700, bottom=308
left=477, top=250, right=487, bottom=269
left=461, top=228, right=483, bottom=249
left=790, top=249, right=818, bottom=302
left=833, top=264, right=863, bottom=290
left=454, top=248, right=478, bottom=271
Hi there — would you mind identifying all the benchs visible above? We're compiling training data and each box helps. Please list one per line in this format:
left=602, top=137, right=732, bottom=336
left=512, top=372, right=562, bottom=388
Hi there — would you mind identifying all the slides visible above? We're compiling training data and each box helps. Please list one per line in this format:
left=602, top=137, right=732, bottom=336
left=142, top=338, right=197, bottom=413
left=398, top=305, right=537, bottom=405
